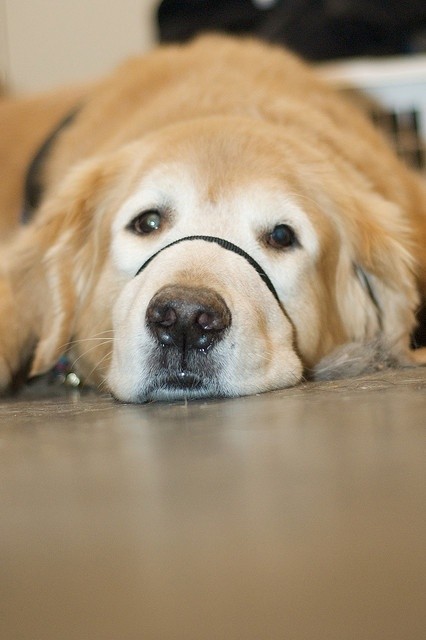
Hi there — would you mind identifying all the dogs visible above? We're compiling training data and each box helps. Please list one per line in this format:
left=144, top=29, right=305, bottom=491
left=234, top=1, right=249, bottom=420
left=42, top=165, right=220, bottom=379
left=0, top=26, right=426, bottom=406
